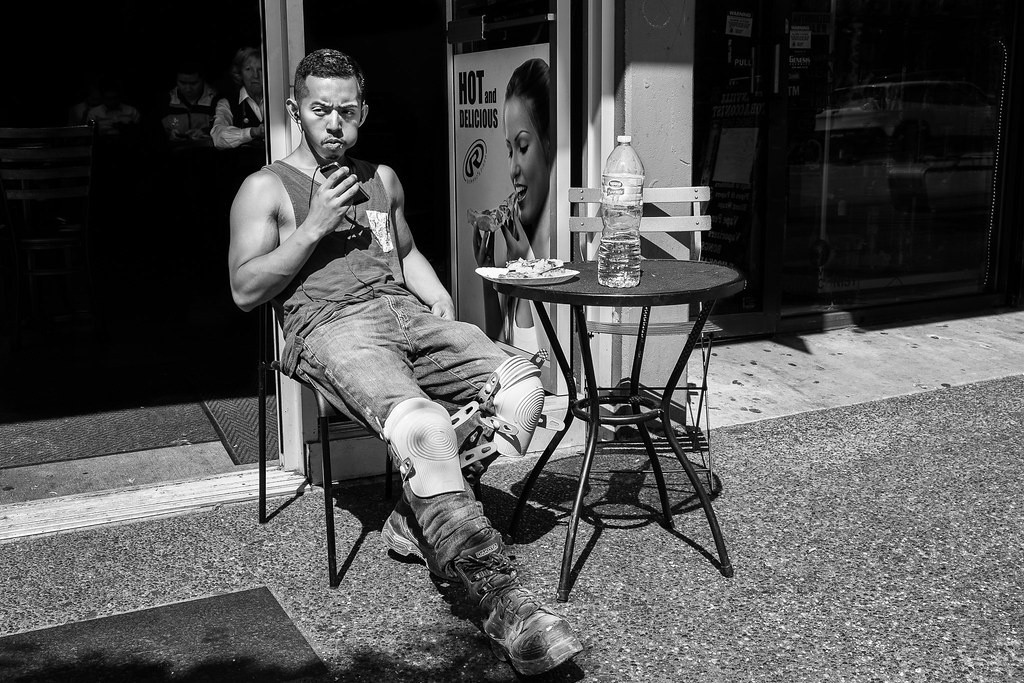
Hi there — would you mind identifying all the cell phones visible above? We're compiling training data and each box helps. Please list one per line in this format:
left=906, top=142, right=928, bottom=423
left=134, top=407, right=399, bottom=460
left=320, top=162, right=370, bottom=205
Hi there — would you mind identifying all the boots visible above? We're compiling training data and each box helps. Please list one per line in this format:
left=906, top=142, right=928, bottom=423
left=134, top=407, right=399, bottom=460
left=381, top=499, right=516, bottom=583
left=453, top=533, right=584, bottom=676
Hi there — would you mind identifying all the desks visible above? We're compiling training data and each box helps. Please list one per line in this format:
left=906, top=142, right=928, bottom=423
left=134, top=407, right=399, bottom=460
left=492, top=261, right=739, bottom=602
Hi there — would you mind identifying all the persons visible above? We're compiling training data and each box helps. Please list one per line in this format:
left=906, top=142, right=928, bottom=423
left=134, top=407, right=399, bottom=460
left=89, top=48, right=264, bottom=151
left=473, top=58, right=559, bottom=361
left=228, top=48, right=585, bottom=677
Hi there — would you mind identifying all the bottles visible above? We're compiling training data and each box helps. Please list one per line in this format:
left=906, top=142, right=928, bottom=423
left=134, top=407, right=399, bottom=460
left=596, top=135, right=644, bottom=288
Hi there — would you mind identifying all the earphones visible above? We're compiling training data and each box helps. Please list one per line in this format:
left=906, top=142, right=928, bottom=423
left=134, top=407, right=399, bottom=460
left=294, top=111, right=300, bottom=119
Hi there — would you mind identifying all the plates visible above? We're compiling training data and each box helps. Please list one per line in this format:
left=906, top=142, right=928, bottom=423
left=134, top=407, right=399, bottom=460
left=475, top=265, right=580, bottom=285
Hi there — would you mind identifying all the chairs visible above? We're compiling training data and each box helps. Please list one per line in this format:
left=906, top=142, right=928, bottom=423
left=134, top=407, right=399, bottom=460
left=0, top=118, right=105, bottom=355
left=259, top=299, right=473, bottom=587
left=568, top=187, right=715, bottom=492
